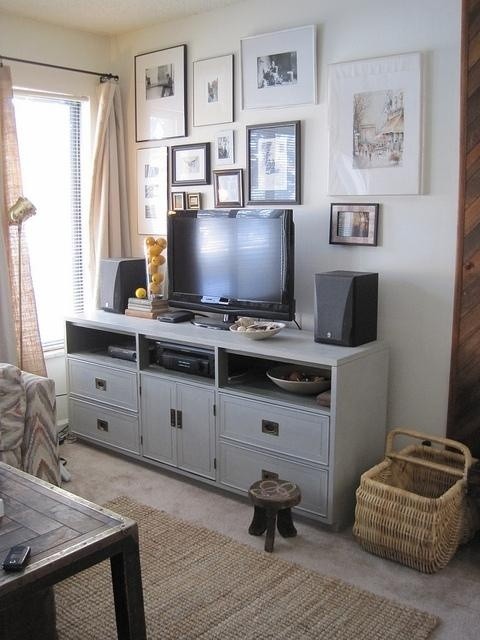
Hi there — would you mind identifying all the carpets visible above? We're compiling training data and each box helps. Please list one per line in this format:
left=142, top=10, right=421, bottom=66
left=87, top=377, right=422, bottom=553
left=52, top=495, right=442, bottom=639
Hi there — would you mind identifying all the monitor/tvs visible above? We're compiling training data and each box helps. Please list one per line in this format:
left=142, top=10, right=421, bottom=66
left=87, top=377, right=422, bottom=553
left=167, top=209, right=295, bottom=330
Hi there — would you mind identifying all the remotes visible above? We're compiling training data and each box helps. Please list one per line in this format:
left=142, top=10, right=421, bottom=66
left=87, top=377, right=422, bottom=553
left=201, top=296, right=230, bottom=305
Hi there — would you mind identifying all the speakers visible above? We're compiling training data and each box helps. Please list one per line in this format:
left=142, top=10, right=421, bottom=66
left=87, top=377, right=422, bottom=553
left=314, top=271, right=379, bottom=348
left=98, top=259, right=146, bottom=314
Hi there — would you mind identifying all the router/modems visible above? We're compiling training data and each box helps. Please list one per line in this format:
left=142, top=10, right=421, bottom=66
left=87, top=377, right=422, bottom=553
left=157, top=310, right=191, bottom=323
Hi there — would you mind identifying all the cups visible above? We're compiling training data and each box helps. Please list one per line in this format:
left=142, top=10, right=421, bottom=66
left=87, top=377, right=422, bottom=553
left=143, top=236, right=166, bottom=301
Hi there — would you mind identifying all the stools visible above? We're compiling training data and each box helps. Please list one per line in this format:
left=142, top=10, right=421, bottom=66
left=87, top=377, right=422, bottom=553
left=248, top=479, right=302, bottom=553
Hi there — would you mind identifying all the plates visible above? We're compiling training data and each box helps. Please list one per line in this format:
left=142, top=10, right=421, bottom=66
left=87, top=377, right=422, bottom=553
left=265, top=363, right=332, bottom=396
left=228, top=320, right=288, bottom=341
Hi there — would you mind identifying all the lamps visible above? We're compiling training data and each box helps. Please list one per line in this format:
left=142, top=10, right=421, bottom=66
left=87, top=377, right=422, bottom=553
left=7, top=196, right=37, bottom=370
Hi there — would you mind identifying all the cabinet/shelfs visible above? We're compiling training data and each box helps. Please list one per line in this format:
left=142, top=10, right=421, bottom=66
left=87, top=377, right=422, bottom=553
left=65, top=319, right=142, bottom=457
left=218, top=346, right=332, bottom=518
left=138, top=333, right=217, bottom=482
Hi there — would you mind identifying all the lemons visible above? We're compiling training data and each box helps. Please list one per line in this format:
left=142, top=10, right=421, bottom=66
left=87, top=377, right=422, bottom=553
left=159, top=255, right=165, bottom=265
left=135, top=288, right=146, bottom=298
left=152, top=273, right=162, bottom=282
left=145, top=237, right=155, bottom=246
left=150, top=282, right=160, bottom=294
left=156, top=238, right=166, bottom=249
left=150, top=246, right=161, bottom=255
left=152, top=256, right=160, bottom=266
left=149, top=264, right=156, bottom=273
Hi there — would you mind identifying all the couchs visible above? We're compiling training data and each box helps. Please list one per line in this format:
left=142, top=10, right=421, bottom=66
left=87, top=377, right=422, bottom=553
left=0, top=363, right=62, bottom=488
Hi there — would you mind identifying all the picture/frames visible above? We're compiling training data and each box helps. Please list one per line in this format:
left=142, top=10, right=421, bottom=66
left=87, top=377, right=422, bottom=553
left=329, top=203, right=380, bottom=247
left=136, top=145, right=169, bottom=235
left=192, top=53, right=234, bottom=128
left=170, top=141, right=211, bottom=187
left=215, top=129, right=235, bottom=165
left=134, top=44, right=189, bottom=143
left=245, top=119, right=301, bottom=205
left=186, top=192, right=202, bottom=210
left=239, top=24, right=318, bottom=113
left=171, top=191, right=185, bottom=211
left=213, top=168, right=244, bottom=208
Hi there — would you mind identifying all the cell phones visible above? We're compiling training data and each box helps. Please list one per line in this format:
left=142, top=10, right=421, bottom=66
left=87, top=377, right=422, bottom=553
left=4, top=545, right=31, bottom=571
